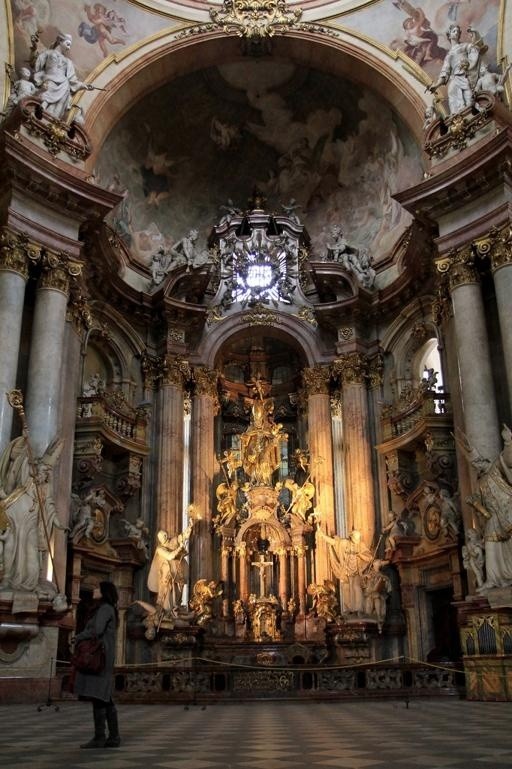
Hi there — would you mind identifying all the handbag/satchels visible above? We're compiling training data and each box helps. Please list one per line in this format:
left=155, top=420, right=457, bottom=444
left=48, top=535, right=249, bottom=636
left=74, top=641, right=107, bottom=674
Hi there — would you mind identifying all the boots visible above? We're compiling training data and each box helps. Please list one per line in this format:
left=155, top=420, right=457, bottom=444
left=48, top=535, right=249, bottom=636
left=80, top=708, right=106, bottom=748
left=106, top=706, right=120, bottom=747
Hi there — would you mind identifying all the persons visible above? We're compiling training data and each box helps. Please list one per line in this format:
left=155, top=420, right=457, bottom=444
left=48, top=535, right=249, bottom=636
left=71, top=581, right=119, bottom=748
